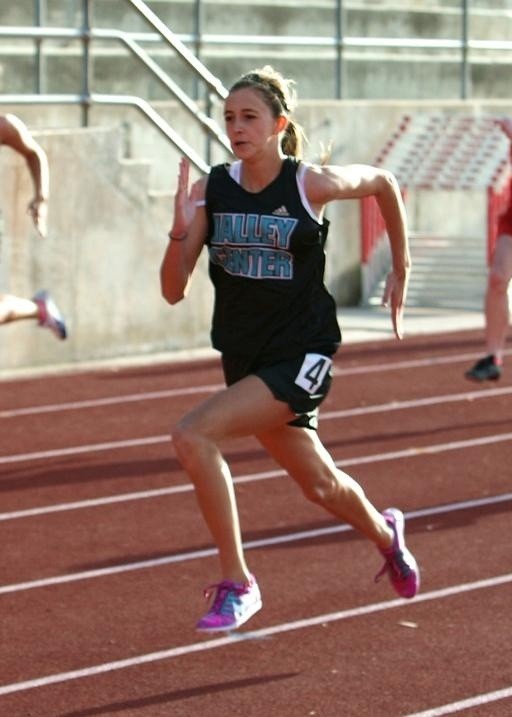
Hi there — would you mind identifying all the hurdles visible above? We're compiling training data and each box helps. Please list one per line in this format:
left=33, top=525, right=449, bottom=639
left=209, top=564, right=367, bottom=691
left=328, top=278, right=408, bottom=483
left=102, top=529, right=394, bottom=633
left=362, top=112, right=512, bottom=311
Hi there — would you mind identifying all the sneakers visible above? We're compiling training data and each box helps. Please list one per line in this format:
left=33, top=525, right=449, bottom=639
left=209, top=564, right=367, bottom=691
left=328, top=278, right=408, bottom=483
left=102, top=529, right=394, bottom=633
left=376, top=506, right=421, bottom=599
left=32, top=289, right=69, bottom=341
left=461, top=351, right=502, bottom=384
left=193, top=573, right=265, bottom=633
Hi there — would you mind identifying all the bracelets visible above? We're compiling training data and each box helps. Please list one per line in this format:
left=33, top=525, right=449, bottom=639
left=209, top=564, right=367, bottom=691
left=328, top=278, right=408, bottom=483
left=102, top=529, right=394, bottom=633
left=167, top=229, right=188, bottom=241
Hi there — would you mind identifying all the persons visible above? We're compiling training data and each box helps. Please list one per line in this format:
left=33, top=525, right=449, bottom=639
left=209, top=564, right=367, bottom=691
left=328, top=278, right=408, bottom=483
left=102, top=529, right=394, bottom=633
left=159, top=65, right=421, bottom=634
left=462, top=119, right=512, bottom=381
left=0, top=109, right=68, bottom=342
